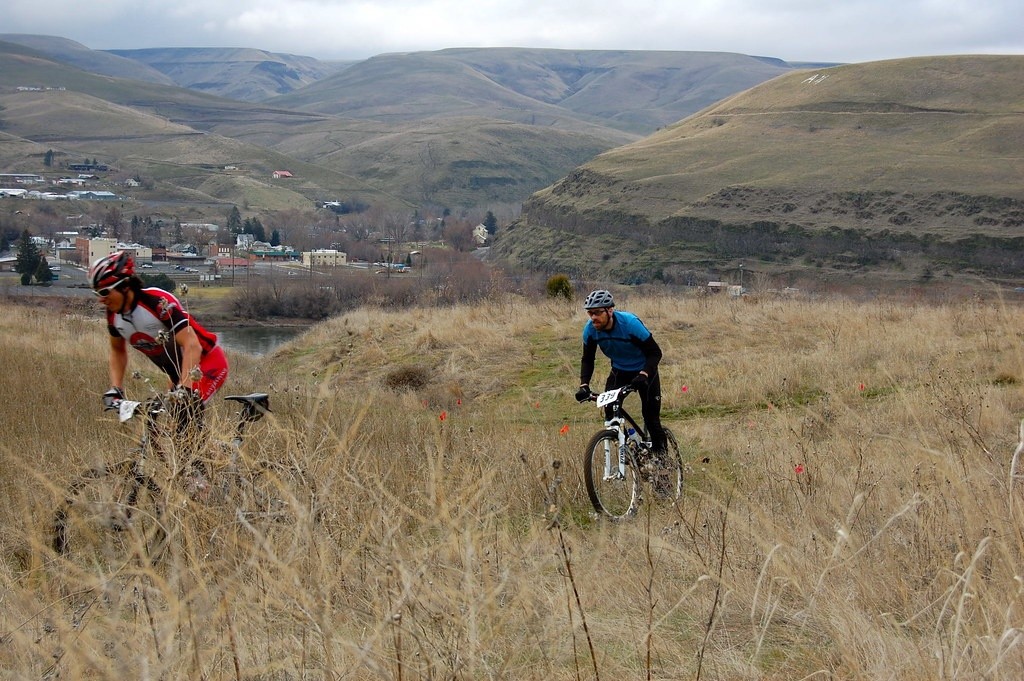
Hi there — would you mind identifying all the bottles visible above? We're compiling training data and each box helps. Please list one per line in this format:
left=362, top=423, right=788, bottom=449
left=162, top=431, right=175, bottom=466
left=627, top=428, right=640, bottom=447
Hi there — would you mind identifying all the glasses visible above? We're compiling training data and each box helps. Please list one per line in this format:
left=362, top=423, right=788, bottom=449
left=586, top=308, right=610, bottom=318
left=92, top=277, right=130, bottom=298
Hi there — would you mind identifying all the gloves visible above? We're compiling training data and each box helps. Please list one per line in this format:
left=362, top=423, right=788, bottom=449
left=103, top=386, right=123, bottom=407
left=163, top=388, right=188, bottom=411
left=631, top=375, right=648, bottom=392
left=574, top=384, right=592, bottom=402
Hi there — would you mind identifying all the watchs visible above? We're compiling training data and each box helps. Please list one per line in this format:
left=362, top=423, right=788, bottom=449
left=176, top=384, right=191, bottom=393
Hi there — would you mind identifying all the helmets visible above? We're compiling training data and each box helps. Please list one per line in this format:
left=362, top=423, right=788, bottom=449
left=86, top=250, right=134, bottom=291
left=583, top=290, right=613, bottom=309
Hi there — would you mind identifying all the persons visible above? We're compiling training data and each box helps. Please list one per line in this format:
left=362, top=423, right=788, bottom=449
left=575, top=290, right=673, bottom=493
left=88, top=250, right=228, bottom=493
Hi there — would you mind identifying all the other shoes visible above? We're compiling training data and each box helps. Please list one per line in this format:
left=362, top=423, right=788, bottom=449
left=656, top=474, right=670, bottom=495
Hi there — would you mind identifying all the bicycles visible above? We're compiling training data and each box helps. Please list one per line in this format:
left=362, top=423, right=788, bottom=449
left=578, top=380, right=685, bottom=526
left=52, top=389, right=315, bottom=616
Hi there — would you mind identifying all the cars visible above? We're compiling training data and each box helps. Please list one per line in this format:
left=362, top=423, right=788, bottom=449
left=167, top=263, right=198, bottom=274
left=136, top=260, right=153, bottom=270
left=373, top=263, right=411, bottom=275
left=49, top=264, right=61, bottom=272
